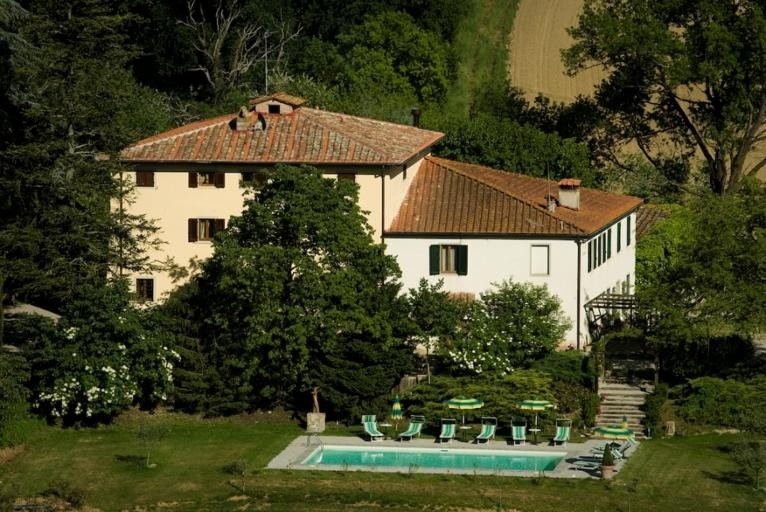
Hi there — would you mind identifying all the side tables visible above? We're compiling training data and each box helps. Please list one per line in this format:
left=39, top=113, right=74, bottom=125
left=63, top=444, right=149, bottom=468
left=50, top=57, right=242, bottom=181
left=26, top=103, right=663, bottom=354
left=460, top=427, right=472, bottom=442
left=529, top=429, right=541, bottom=445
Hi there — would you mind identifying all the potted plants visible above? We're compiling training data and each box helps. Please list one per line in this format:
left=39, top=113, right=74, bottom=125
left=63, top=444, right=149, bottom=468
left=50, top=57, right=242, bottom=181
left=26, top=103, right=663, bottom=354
left=600, top=443, right=616, bottom=479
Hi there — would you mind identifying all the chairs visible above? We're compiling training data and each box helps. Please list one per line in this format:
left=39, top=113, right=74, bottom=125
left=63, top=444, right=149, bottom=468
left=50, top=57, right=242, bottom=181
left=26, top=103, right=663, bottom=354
left=439, top=419, right=457, bottom=444
left=476, top=417, right=497, bottom=444
left=590, top=439, right=631, bottom=462
left=573, top=461, right=602, bottom=471
left=361, top=415, right=384, bottom=442
left=399, top=415, right=425, bottom=442
left=553, top=419, right=572, bottom=447
left=511, top=416, right=527, bottom=446
